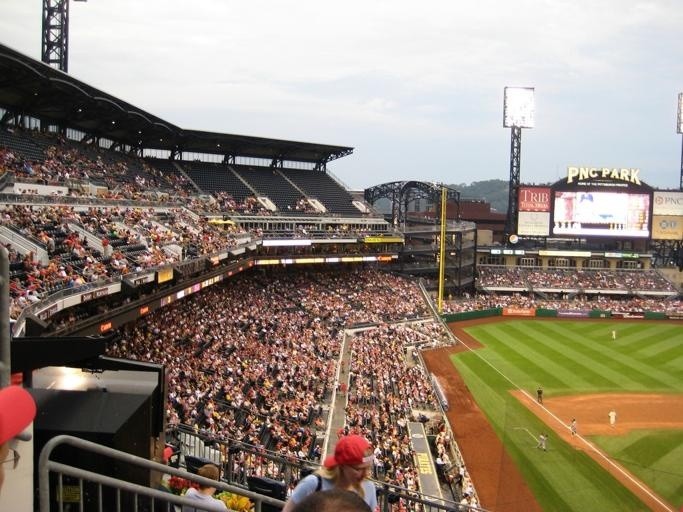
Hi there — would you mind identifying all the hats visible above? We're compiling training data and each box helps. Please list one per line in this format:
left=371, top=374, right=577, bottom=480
left=162, top=447, right=172, bottom=460
left=324, top=435, right=374, bottom=467
left=0, top=385, right=36, bottom=445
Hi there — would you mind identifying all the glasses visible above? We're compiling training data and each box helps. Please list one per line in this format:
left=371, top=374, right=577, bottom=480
left=0, top=448, right=19, bottom=469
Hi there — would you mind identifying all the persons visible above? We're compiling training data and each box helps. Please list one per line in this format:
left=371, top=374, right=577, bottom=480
left=608, top=409, right=615, bottom=428
left=479, top=266, right=682, bottom=315
left=0, top=126, right=480, bottom=512
left=536, top=387, right=543, bottom=402
left=537, top=433, right=548, bottom=452
left=610, top=329, right=615, bottom=339
left=571, top=418, right=576, bottom=437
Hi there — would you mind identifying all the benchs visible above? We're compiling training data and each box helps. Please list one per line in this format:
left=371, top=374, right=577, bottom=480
left=0, top=124, right=483, bottom=512
